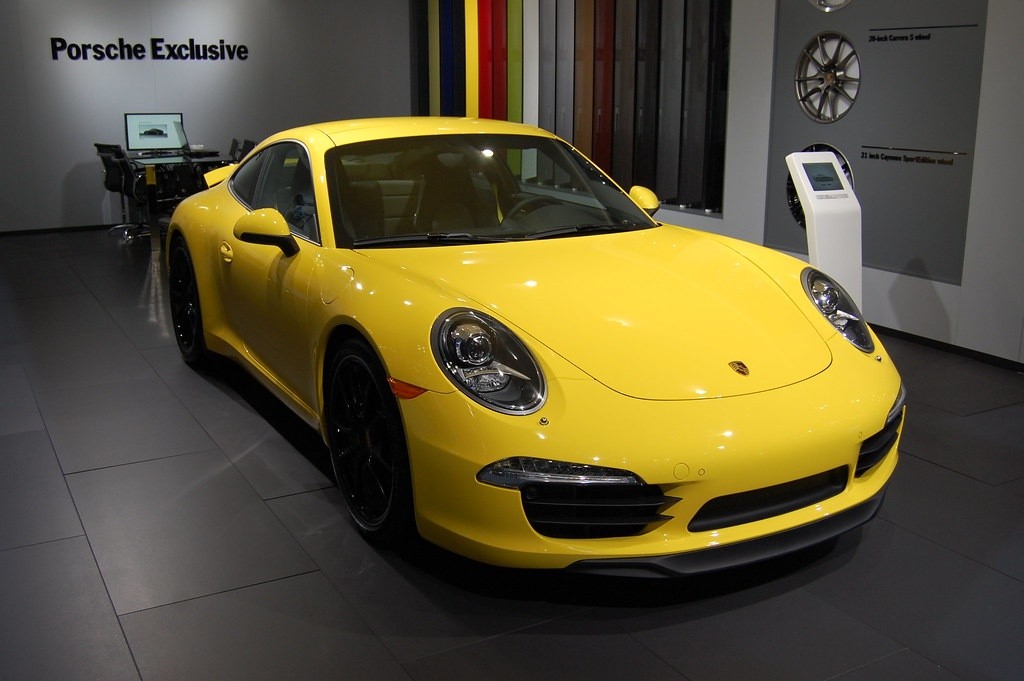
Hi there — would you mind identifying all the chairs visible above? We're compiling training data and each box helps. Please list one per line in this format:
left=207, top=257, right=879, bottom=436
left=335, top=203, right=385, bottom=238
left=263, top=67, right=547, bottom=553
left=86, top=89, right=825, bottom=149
left=93, top=139, right=258, bottom=248
left=290, top=154, right=517, bottom=242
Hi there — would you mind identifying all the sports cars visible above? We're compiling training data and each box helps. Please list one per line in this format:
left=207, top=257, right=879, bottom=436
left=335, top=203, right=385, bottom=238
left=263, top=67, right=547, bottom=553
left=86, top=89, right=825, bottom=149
left=164, top=116, right=911, bottom=581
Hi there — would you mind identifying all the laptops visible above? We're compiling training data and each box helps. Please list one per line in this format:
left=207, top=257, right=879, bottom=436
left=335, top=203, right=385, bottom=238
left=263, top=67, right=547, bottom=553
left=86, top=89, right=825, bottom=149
left=173, top=120, right=219, bottom=156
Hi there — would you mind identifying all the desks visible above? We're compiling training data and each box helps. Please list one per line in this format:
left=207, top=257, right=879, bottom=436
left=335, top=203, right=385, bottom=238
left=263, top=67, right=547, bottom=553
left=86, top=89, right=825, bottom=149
left=131, top=154, right=236, bottom=251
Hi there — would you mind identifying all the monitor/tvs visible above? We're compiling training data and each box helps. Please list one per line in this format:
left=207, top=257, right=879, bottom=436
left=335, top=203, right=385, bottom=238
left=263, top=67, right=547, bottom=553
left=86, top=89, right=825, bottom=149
left=125, top=112, right=184, bottom=154
